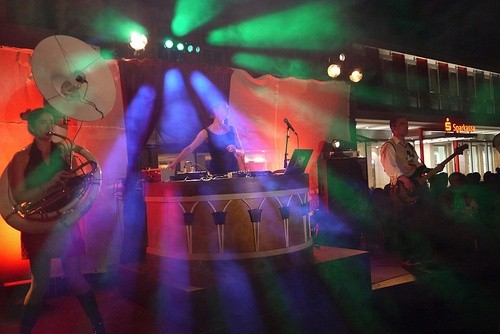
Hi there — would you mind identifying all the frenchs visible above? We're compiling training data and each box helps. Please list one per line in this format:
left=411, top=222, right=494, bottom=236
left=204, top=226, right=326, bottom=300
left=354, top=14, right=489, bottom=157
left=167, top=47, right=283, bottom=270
left=0, top=131, right=104, bottom=235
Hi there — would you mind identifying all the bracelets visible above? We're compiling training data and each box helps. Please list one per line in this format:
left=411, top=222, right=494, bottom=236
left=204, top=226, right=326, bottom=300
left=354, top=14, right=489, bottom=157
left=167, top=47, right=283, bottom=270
left=234, top=149, right=237, bottom=157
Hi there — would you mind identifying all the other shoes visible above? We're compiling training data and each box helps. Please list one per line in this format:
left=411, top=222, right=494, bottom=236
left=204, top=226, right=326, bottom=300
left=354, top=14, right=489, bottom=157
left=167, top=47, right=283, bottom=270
left=401, top=256, right=416, bottom=265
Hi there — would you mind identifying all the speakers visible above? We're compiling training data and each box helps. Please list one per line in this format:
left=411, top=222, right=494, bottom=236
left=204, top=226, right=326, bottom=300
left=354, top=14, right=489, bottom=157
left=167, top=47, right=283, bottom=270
left=327, top=157, right=369, bottom=249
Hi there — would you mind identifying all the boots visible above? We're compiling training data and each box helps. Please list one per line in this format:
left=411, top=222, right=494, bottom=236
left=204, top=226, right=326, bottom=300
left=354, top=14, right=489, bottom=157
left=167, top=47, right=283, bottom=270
left=19, top=302, right=45, bottom=334
left=74, top=289, right=107, bottom=334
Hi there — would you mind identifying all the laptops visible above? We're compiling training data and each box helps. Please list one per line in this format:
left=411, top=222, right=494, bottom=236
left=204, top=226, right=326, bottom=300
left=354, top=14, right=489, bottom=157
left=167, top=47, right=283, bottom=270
left=271, top=148, right=314, bottom=176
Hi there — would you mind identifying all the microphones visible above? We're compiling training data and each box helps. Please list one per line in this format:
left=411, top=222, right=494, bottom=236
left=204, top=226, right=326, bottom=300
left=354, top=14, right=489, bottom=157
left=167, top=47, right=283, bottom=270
left=284, top=118, right=298, bottom=135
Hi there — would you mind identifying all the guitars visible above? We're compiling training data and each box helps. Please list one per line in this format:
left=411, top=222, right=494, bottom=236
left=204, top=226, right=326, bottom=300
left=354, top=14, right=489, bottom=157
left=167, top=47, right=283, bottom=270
left=390, top=144, right=468, bottom=210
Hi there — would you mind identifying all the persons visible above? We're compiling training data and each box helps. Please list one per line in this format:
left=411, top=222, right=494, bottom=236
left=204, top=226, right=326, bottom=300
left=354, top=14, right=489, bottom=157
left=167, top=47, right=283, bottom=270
left=12, top=108, right=105, bottom=334
left=368, top=133, right=500, bottom=259
left=380, top=115, right=445, bottom=268
left=164, top=101, right=244, bottom=174
